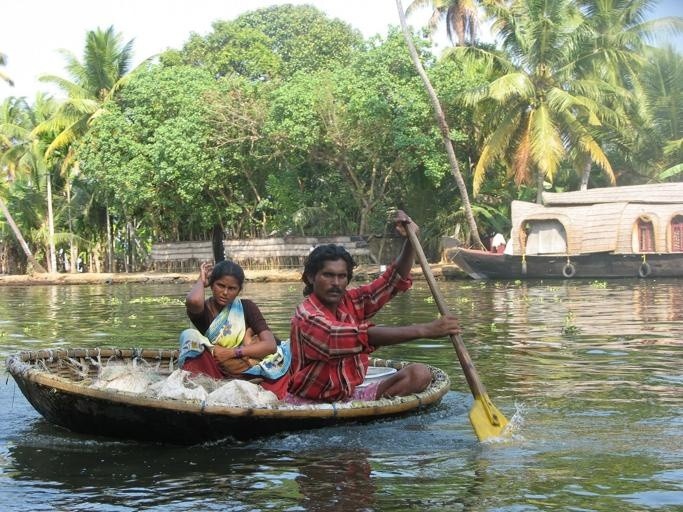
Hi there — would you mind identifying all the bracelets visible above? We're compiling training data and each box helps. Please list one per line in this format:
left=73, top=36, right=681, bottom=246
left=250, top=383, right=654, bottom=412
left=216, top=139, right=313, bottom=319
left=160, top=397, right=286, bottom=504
left=234, top=346, right=244, bottom=358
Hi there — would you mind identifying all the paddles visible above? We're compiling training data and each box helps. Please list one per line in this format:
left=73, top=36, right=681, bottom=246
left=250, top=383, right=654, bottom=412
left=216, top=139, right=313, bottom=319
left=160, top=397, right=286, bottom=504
left=402, top=221, right=525, bottom=443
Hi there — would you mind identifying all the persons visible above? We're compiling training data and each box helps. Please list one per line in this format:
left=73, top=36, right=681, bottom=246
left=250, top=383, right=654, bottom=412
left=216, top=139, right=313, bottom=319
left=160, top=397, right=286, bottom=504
left=283, top=210, right=463, bottom=409
left=177, top=222, right=288, bottom=400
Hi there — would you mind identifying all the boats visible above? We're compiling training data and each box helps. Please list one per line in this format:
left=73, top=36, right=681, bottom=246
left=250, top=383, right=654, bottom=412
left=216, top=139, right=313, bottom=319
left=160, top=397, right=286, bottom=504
left=441, top=181, right=683, bottom=287
left=7, top=345, right=453, bottom=448
